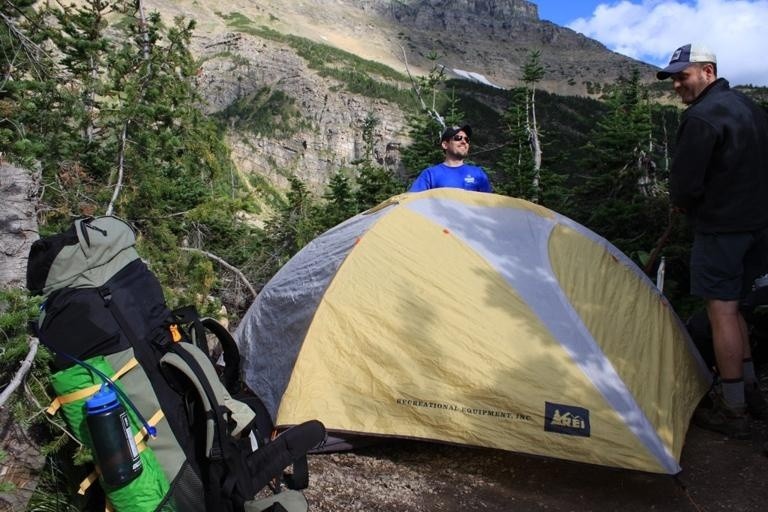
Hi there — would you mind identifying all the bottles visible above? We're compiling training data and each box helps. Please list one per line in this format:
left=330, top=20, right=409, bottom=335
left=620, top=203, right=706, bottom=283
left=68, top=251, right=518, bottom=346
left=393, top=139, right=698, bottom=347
left=86, top=382, right=143, bottom=486
left=656, top=256, right=666, bottom=297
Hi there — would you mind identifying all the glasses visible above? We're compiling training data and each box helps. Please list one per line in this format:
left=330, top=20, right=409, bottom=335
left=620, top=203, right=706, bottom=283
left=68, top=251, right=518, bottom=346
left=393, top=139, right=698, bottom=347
left=449, top=135, right=470, bottom=144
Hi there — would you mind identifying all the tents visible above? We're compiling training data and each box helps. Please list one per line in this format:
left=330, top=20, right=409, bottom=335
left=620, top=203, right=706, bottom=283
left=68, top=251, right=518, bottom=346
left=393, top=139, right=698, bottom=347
left=208, top=184, right=715, bottom=481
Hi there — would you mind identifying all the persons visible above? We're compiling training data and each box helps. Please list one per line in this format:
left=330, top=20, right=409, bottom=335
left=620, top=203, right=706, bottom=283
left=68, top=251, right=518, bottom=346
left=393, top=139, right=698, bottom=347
left=403, top=124, right=493, bottom=195
left=654, top=42, right=767, bottom=441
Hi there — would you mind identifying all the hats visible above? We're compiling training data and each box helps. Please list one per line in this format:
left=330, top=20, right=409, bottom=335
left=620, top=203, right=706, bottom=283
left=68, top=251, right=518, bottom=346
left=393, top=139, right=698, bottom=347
left=442, top=125, right=471, bottom=141
left=657, top=44, right=717, bottom=80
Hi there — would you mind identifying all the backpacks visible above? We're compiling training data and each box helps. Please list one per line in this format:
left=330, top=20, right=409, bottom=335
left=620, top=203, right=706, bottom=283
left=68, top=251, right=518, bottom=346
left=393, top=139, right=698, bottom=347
left=26, top=215, right=328, bottom=512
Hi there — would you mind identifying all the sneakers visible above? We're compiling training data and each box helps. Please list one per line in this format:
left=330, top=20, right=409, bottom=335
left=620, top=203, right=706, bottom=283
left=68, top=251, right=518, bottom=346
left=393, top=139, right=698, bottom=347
left=692, top=381, right=761, bottom=439
